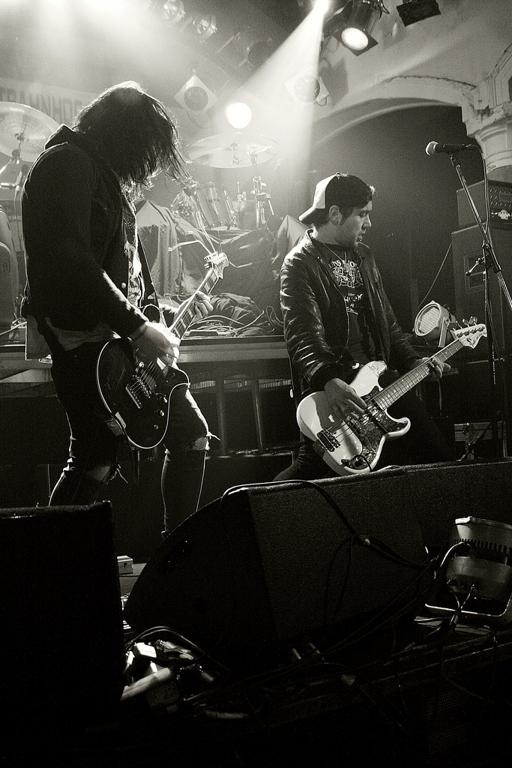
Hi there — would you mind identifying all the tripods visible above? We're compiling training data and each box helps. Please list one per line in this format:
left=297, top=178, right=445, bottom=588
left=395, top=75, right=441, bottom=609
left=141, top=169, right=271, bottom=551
left=447, top=153, right=512, bottom=461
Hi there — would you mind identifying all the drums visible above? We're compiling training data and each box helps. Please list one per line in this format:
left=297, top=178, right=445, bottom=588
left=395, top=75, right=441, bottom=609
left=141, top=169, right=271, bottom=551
left=179, top=183, right=239, bottom=235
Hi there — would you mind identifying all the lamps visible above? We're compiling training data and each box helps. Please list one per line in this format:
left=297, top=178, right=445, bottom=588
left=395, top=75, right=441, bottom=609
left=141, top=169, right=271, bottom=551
left=331, top=0, right=390, bottom=57
left=425, top=514, right=512, bottom=621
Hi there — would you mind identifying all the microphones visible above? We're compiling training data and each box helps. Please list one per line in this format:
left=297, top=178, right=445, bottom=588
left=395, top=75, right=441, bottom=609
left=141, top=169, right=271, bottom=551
left=425, top=141, right=480, bottom=156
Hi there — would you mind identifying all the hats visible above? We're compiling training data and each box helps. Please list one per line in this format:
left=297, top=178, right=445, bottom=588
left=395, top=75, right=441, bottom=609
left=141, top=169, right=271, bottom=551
left=298, top=173, right=372, bottom=224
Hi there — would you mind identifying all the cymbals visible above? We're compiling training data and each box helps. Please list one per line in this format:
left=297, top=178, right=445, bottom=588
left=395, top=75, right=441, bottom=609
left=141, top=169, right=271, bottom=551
left=1, top=102, right=62, bottom=163
left=192, top=133, right=284, bottom=169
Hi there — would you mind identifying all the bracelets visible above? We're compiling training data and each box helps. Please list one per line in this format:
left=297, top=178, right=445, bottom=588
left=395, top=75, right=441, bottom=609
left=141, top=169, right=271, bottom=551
left=133, top=326, right=149, bottom=342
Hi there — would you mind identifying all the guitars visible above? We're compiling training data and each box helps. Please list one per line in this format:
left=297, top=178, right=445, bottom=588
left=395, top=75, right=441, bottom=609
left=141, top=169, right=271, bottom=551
left=82, top=252, right=227, bottom=448
left=294, top=316, right=491, bottom=474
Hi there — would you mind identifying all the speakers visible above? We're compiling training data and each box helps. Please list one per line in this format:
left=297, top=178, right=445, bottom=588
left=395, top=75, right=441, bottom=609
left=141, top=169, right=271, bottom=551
left=0, top=499, right=127, bottom=720
left=123, top=459, right=511, bottom=713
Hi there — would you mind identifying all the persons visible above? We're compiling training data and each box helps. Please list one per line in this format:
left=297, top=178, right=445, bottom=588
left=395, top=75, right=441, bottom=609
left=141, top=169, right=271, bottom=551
left=273, top=170, right=453, bottom=479
left=19, top=84, right=213, bottom=537
